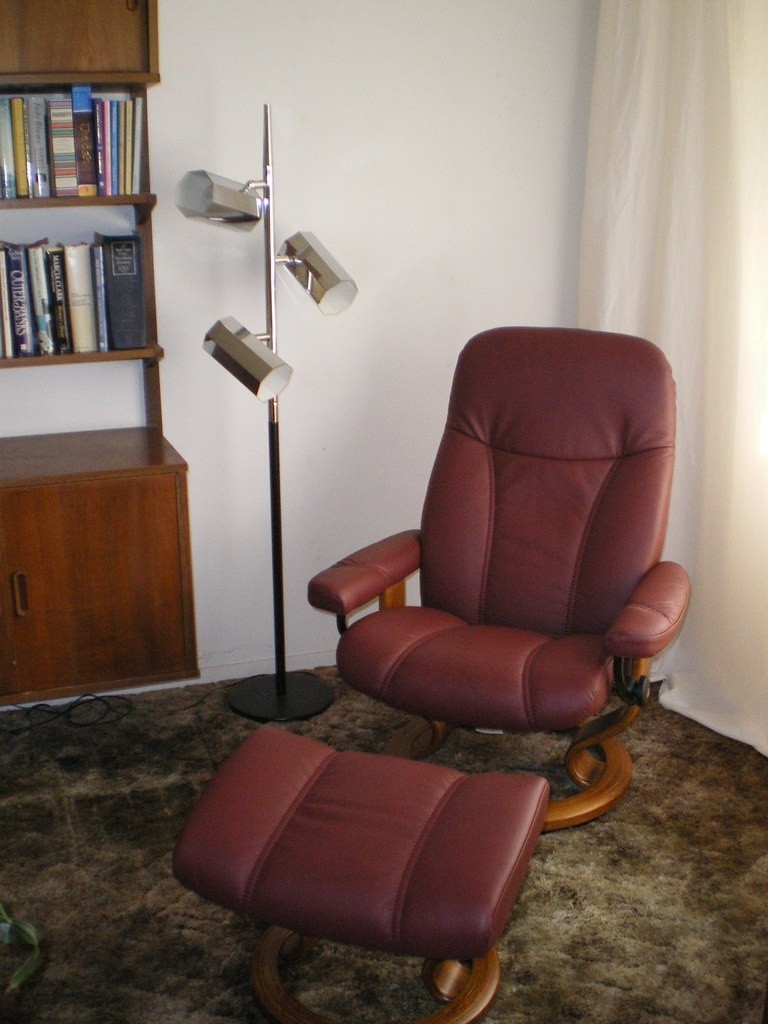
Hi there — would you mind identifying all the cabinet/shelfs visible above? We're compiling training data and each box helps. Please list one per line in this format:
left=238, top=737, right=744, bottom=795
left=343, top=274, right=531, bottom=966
left=0, top=0, right=203, bottom=707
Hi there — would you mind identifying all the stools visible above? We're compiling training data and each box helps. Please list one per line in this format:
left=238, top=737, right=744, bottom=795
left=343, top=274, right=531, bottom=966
left=169, top=725, right=552, bottom=1024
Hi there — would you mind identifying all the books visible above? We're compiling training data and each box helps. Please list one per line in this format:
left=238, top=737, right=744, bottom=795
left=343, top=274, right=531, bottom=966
left=0, top=85, right=143, bottom=358
left=95, top=230, right=148, bottom=351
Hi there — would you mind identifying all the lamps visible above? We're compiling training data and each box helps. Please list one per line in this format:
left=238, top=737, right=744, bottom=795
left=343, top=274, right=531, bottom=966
left=173, top=105, right=359, bottom=725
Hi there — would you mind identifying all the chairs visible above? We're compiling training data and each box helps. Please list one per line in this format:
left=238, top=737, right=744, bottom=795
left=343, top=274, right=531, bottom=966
left=306, top=328, right=693, bottom=835
